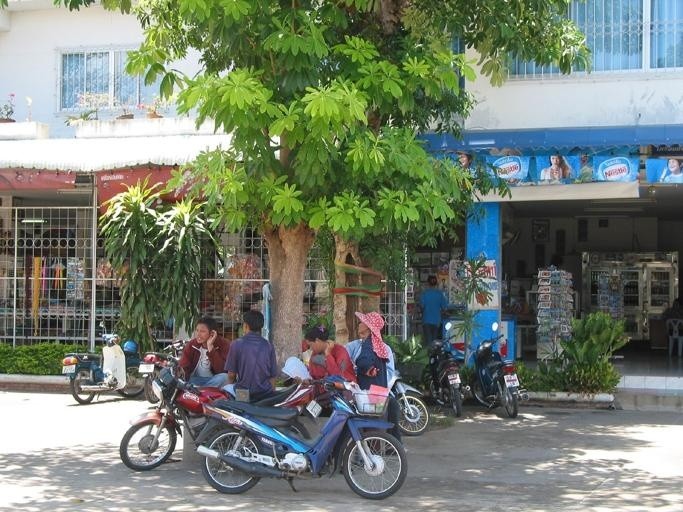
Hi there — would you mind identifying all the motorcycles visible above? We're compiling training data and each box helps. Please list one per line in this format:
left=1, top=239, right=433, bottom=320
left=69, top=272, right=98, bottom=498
left=469, top=321, right=528, bottom=418
left=416, top=320, right=470, bottom=418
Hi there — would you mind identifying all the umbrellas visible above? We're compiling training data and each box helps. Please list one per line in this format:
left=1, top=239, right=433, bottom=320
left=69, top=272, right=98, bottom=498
left=41, top=256, right=65, bottom=299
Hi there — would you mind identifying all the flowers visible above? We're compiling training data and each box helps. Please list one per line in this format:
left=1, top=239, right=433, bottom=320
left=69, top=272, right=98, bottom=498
left=0, top=92, right=16, bottom=119
left=136, top=95, right=164, bottom=113
left=76, top=91, right=134, bottom=114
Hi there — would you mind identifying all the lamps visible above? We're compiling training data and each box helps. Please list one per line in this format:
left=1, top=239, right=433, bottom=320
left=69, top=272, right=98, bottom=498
left=575, top=198, right=658, bottom=219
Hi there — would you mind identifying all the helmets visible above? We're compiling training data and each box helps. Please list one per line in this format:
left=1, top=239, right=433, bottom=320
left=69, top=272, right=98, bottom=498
left=124, top=339, right=138, bottom=353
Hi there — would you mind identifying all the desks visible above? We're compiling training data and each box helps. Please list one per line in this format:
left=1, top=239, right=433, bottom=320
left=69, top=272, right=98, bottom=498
left=515, top=324, right=537, bottom=358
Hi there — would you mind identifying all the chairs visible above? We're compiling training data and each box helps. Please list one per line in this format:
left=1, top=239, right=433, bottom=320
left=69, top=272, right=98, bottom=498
left=665, top=318, right=683, bottom=357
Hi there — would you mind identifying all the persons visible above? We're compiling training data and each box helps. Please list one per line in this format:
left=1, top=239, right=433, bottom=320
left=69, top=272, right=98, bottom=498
left=224, top=309, right=277, bottom=404
left=305, top=326, right=357, bottom=417
left=540, top=156, right=570, bottom=180
left=662, top=298, right=683, bottom=337
left=418, top=275, right=448, bottom=346
left=343, top=311, right=408, bottom=455
left=175, top=314, right=231, bottom=389
left=659, top=158, right=683, bottom=184
left=458, top=154, right=477, bottom=178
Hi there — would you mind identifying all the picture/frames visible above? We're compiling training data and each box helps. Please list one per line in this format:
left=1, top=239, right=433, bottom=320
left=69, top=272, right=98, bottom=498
left=531, top=219, right=550, bottom=242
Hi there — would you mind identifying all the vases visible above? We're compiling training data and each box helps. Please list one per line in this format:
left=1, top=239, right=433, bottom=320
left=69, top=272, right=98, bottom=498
left=115, top=114, right=134, bottom=119
left=145, top=113, right=163, bottom=118
left=0, top=118, right=16, bottom=124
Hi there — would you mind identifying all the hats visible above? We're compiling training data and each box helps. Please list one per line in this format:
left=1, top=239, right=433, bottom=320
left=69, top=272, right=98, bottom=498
left=354, top=310, right=385, bottom=341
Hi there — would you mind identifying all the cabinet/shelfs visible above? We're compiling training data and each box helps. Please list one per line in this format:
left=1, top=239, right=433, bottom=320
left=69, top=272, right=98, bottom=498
left=534, top=266, right=574, bottom=360
